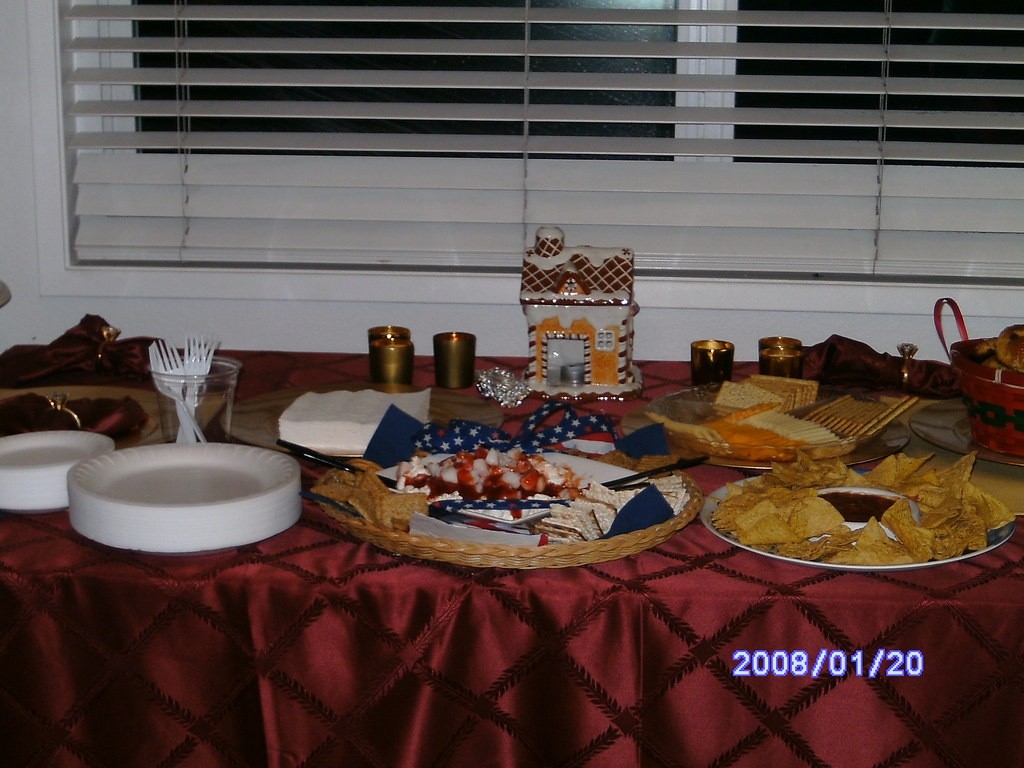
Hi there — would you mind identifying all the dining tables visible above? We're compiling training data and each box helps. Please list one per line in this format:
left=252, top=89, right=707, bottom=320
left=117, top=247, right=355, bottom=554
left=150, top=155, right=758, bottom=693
left=1, top=347, right=1024, bottom=768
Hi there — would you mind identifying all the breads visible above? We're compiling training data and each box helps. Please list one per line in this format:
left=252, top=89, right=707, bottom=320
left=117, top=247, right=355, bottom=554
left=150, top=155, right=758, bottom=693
left=974, top=322, right=1024, bottom=374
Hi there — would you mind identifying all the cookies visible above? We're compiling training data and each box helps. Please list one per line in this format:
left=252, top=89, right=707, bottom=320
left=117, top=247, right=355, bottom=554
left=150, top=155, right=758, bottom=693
left=527, top=474, right=690, bottom=544
left=644, top=373, right=918, bottom=462
left=308, top=457, right=429, bottom=532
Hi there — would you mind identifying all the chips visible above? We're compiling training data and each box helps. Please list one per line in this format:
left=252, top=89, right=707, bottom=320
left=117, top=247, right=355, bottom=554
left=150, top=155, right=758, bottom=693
left=711, top=450, right=1017, bottom=566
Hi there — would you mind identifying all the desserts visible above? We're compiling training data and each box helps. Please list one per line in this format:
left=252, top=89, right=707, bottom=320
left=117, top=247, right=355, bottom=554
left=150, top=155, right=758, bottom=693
left=397, top=447, right=577, bottom=519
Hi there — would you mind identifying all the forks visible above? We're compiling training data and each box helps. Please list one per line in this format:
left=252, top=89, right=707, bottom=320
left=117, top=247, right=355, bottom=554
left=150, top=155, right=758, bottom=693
left=149, top=336, right=220, bottom=442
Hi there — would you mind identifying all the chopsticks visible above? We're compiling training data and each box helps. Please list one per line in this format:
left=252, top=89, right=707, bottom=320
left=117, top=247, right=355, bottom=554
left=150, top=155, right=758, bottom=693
left=601, top=456, right=709, bottom=491
left=277, top=439, right=397, bottom=488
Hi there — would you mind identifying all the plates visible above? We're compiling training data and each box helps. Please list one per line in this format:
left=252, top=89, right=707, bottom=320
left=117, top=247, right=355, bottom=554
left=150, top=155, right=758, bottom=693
left=646, top=383, right=886, bottom=460
left=69, top=442, right=302, bottom=554
left=699, top=476, right=1016, bottom=572
left=620, top=386, right=910, bottom=469
left=0, top=430, right=116, bottom=514
left=218, top=382, right=504, bottom=457
left=909, top=395, right=1024, bottom=466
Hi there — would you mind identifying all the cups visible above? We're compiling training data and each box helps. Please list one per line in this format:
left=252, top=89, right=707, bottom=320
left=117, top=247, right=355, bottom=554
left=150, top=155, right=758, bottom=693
left=758, top=338, right=803, bottom=378
left=368, top=326, right=411, bottom=380
left=369, top=338, right=415, bottom=385
left=434, top=332, right=476, bottom=388
left=147, top=355, right=243, bottom=443
left=691, top=340, right=734, bottom=384
